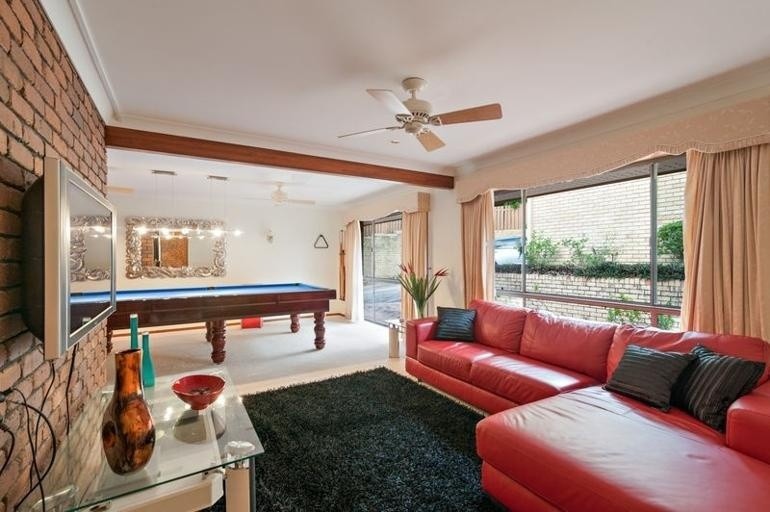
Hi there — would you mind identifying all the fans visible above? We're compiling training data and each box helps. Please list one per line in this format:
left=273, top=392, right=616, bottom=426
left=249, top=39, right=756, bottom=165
left=238, top=182, right=317, bottom=206
left=336, top=77, right=502, bottom=153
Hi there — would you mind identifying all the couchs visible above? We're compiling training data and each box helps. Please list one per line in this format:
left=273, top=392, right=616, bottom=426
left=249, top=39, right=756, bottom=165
left=405, top=298, right=770, bottom=512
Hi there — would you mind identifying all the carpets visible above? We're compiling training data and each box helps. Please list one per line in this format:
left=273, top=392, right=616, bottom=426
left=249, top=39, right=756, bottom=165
left=165, top=366, right=508, bottom=512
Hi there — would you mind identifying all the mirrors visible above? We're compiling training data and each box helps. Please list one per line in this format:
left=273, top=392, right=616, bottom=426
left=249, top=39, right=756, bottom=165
left=125, top=215, right=229, bottom=280
left=69, top=215, right=112, bottom=283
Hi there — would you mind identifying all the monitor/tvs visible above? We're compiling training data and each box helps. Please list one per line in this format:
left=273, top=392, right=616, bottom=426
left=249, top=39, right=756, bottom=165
left=21, top=156, right=117, bottom=361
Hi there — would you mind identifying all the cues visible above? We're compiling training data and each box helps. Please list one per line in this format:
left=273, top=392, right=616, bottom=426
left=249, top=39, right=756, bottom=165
left=339, top=230, right=345, bottom=301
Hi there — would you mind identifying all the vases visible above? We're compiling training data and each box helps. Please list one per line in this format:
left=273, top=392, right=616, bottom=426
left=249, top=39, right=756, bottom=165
left=102, top=348, right=156, bottom=477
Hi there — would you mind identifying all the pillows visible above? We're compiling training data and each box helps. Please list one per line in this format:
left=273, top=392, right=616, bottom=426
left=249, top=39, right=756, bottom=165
left=602, top=343, right=766, bottom=433
left=431, top=306, right=477, bottom=343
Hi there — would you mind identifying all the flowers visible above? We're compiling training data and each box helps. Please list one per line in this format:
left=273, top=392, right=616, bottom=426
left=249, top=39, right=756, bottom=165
left=391, top=260, right=452, bottom=319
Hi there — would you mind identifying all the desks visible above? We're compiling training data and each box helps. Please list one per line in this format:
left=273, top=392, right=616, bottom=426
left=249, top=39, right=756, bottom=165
left=13, top=364, right=265, bottom=512
left=70, top=291, right=111, bottom=333
left=107, top=282, right=337, bottom=365
